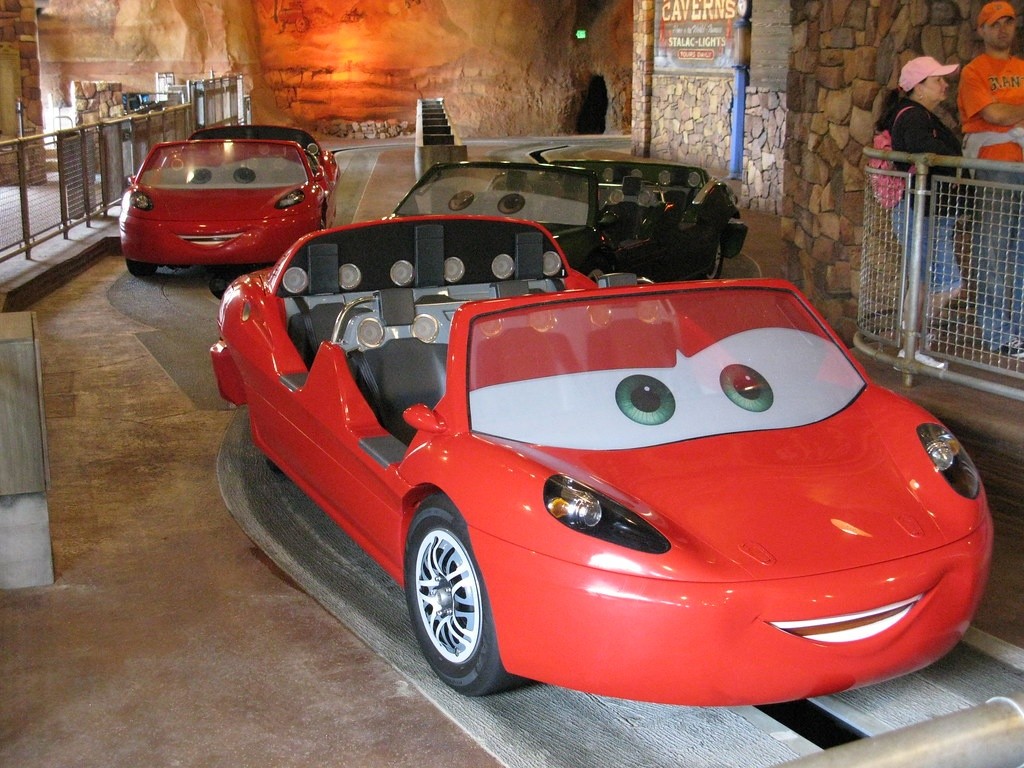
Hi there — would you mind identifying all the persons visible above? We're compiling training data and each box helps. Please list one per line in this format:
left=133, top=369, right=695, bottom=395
left=875, top=56, right=974, bottom=372
left=957, top=2, right=1024, bottom=359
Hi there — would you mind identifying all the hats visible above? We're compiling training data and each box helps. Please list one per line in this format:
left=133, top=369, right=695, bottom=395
left=977, top=0, right=1017, bottom=27
left=897, top=56, right=960, bottom=93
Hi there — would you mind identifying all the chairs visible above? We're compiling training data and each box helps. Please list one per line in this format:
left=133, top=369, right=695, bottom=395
left=288, top=287, right=552, bottom=372
left=354, top=335, right=450, bottom=447
left=599, top=201, right=649, bottom=244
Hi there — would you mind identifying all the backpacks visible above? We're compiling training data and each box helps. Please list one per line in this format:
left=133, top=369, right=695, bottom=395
left=869, top=105, right=916, bottom=209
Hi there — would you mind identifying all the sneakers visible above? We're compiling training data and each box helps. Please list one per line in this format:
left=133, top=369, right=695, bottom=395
left=893, top=350, right=945, bottom=375
left=898, top=321, right=936, bottom=352
left=996, top=337, right=1024, bottom=359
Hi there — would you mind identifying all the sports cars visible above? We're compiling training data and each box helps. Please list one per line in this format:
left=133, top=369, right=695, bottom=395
left=383, top=153, right=749, bottom=284
left=119, top=123, right=341, bottom=278
left=210, top=212, right=994, bottom=708
